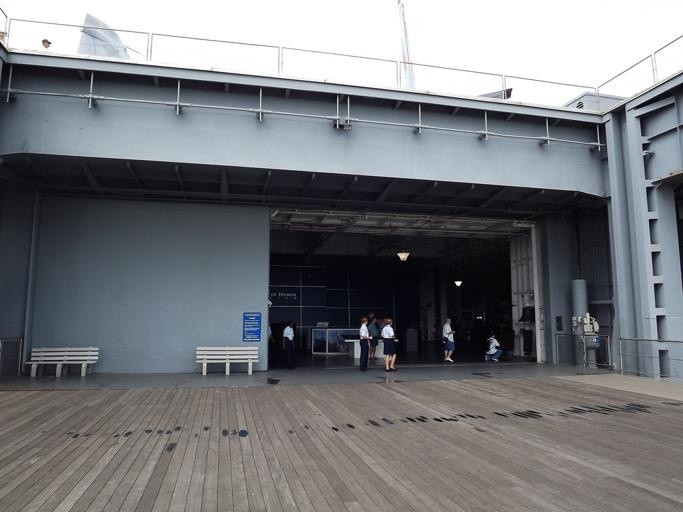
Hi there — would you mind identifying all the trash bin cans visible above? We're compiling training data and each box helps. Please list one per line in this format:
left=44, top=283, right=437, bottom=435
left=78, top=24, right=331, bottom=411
left=0, top=336, right=23, bottom=379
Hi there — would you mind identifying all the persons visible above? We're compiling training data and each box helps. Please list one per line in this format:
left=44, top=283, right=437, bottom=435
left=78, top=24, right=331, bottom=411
left=442, top=318, right=455, bottom=362
left=360, top=315, right=399, bottom=371
left=282, top=322, right=297, bottom=370
left=484, top=333, right=502, bottom=362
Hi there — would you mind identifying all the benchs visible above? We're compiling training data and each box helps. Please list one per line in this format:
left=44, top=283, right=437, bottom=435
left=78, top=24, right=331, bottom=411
left=23, top=347, right=98, bottom=377
left=193, top=346, right=259, bottom=376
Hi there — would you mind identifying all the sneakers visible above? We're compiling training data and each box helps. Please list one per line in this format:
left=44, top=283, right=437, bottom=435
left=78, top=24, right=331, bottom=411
left=360, top=356, right=501, bottom=371
